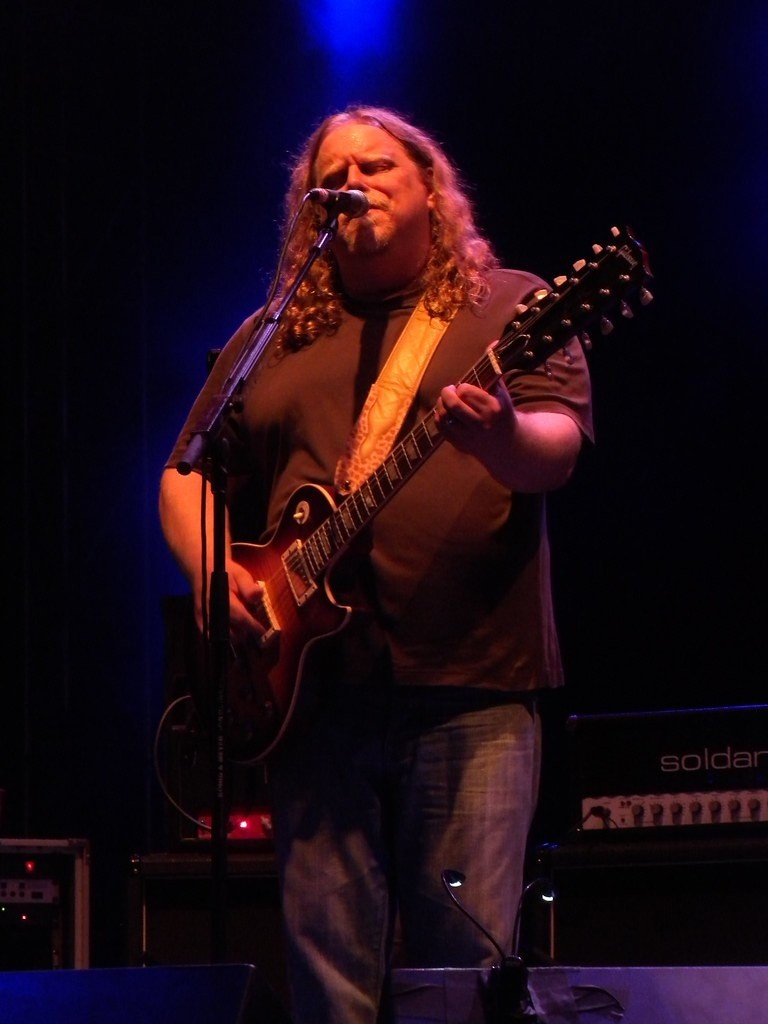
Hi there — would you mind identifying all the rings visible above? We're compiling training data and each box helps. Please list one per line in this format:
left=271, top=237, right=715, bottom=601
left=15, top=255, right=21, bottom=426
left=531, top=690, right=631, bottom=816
left=446, top=418, right=454, bottom=425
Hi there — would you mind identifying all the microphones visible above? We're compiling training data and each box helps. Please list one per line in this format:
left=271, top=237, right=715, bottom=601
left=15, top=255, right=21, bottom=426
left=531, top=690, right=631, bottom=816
left=309, top=188, right=370, bottom=218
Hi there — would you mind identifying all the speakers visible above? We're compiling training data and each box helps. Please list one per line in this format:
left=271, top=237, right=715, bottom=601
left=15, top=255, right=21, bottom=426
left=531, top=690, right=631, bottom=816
left=376, top=965, right=768, bottom=1024
left=549, top=852, right=768, bottom=967
left=0, top=964, right=297, bottom=1024
left=131, top=852, right=303, bottom=974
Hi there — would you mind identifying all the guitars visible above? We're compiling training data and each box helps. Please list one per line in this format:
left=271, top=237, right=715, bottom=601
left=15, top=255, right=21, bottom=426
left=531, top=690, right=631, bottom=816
left=201, top=228, right=654, bottom=771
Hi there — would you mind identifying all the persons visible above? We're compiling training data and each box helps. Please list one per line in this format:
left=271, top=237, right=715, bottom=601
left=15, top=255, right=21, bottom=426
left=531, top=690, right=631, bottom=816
left=159, top=105, right=594, bottom=1024
